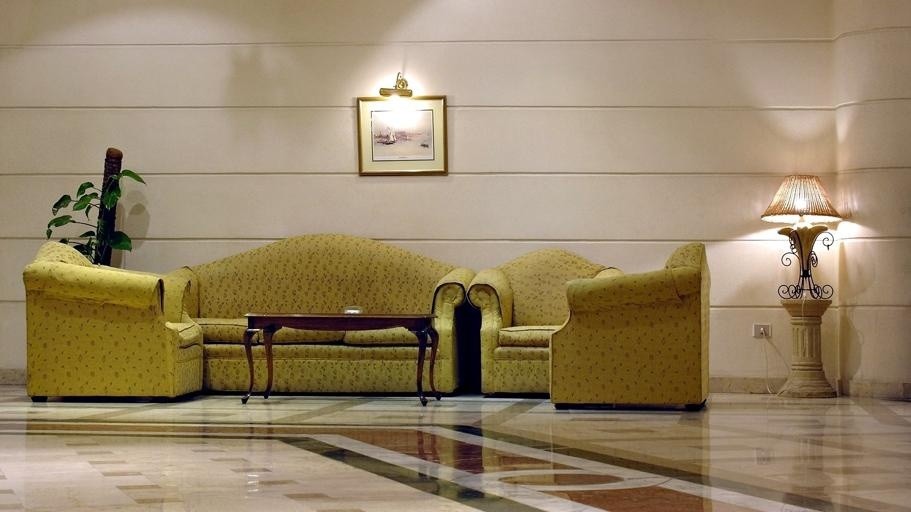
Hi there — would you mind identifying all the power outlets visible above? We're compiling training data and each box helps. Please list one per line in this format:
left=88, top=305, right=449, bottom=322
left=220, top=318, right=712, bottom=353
left=752, top=322, right=773, bottom=339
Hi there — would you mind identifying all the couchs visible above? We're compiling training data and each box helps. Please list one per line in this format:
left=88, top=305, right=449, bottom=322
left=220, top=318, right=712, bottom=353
left=171, top=230, right=471, bottom=393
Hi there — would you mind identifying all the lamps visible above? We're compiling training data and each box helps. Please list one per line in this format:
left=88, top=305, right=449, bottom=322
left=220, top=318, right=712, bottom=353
left=762, top=171, right=847, bottom=301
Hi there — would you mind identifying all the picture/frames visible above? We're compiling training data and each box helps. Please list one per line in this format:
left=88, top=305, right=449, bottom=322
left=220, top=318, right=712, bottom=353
left=356, top=95, right=448, bottom=178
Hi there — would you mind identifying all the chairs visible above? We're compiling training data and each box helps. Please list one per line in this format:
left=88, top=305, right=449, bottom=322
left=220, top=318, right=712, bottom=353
left=22, top=242, right=206, bottom=401
